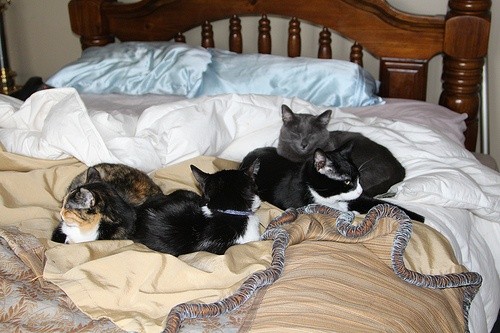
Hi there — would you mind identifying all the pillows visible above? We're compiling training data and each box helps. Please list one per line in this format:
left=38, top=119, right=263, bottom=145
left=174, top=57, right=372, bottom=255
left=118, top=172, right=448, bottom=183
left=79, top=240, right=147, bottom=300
left=44, top=42, right=381, bottom=110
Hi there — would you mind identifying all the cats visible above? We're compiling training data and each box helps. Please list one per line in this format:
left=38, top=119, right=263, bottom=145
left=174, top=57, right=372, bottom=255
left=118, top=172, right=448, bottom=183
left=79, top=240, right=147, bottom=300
left=52, top=103, right=425, bottom=258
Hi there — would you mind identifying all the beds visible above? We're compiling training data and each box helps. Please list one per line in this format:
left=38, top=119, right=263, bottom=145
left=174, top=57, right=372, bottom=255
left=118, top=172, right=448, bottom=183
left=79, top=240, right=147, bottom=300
left=0, top=0, right=500, bottom=333
